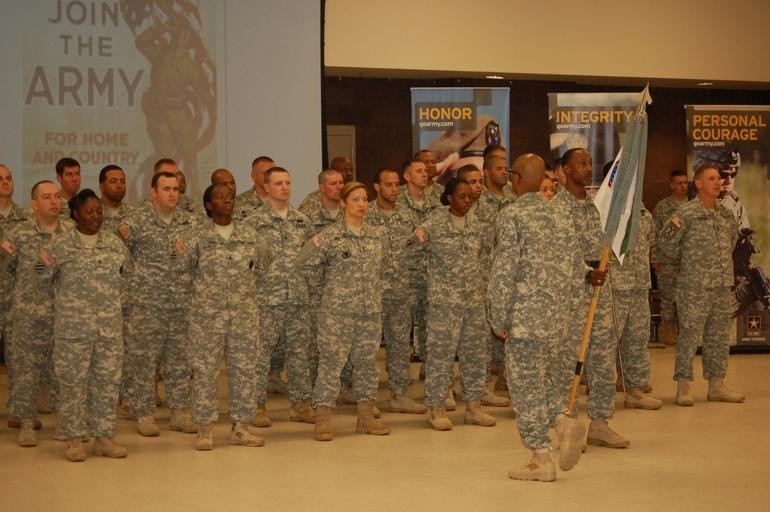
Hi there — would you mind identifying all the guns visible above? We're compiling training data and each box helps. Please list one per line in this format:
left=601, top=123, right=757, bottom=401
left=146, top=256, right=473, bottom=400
left=732, top=228, right=770, bottom=315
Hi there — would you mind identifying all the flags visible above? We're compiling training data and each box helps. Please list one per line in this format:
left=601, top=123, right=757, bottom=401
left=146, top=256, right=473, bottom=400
left=592, top=107, right=650, bottom=268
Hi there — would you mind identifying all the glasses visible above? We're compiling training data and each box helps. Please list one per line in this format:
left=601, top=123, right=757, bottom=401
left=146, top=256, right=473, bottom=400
left=507, top=167, right=522, bottom=179
left=718, top=170, right=737, bottom=180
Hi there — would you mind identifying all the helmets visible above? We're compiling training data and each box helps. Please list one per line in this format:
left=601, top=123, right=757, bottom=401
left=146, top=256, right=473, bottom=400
left=691, top=142, right=740, bottom=173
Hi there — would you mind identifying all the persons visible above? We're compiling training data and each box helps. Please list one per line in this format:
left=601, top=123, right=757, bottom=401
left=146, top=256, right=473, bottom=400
left=657, top=162, right=747, bottom=406
left=485, top=151, right=587, bottom=483
left=692, top=140, right=769, bottom=345
left=650, top=168, right=690, bottom=347
left=1, top=144, right=671, bottom=464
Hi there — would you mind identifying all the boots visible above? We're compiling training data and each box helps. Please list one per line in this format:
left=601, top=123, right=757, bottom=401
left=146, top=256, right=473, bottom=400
left=230, top=403, right=272, bottom=447
left=339, top=389, right=390, bottom=435
left=419, top=354, right=511, bottom=431
left=616, top=376, right=664, bottom=409
left=121, top=400, right=163, bottom=436
left=93, top=437, right=127, bottom=458
left=169, top=410, right=198, bottom=433
left=675, top=377, right=694, bottom=407
left=659, top=321, right=679, bottom=347
left=389, top=395, right=427, bottom=414
left=289, top=400, right=334, bottom=441
left=267, top=378, right=288, bottom=394
left=707, top=377, right=745, bottom=403
left=5, top=375, right=54, bottom=446
left=196, top=418, right=214, bottom=449
left=555, top=415, right=586, bottom=472
left=507, top=449, right=557, bottom=482
left=585, top=417, right=630, bottom=450
left=56, top=433, right=89, bottom=463
